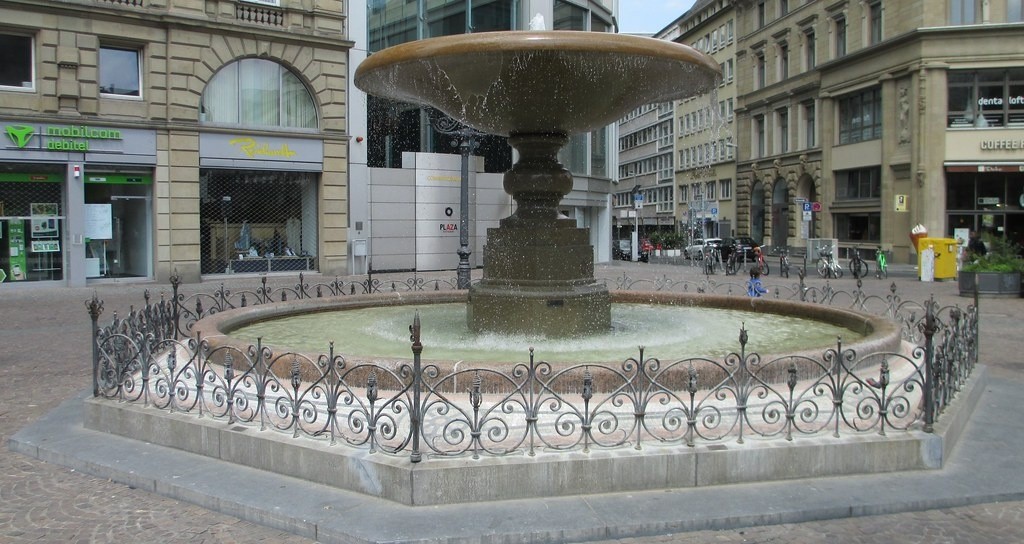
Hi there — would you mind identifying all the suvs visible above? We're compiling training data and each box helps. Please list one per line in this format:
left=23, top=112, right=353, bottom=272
left=683, top=238, right=724, bottom=261
left=720, top=235, right=760, bottom=262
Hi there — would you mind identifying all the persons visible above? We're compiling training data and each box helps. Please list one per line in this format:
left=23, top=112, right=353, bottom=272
left=703, top=240, right=716, bottom=273
left=965, top=232, right=986, bottom=262
left=746, top=266, right=769, bottom=297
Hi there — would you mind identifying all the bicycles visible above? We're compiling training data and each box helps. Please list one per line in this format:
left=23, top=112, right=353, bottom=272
left=849, top=244, right=869, bottom=278
left=814, top=243, right=844, bottom=279
left=703, top=242, right=718, bottom=276
left=771, top=245, right=792, bottom=279
left=751, top=244, right=770, bottom=276
left=725, top=244, right=742, bottom=277
left=874, top=245, right=890, bottom=280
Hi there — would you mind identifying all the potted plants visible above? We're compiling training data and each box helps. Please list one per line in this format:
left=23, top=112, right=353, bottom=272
left=652, top=229, right=682, bottom=256
left=959, top=232, right=1024, bottom=298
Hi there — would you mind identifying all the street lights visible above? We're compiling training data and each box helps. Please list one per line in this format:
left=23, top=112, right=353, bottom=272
left=697, top=193, right=707, bottom=249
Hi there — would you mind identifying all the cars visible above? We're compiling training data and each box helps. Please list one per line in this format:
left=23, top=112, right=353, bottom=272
left=613, top=240, right=649, bottom=263
